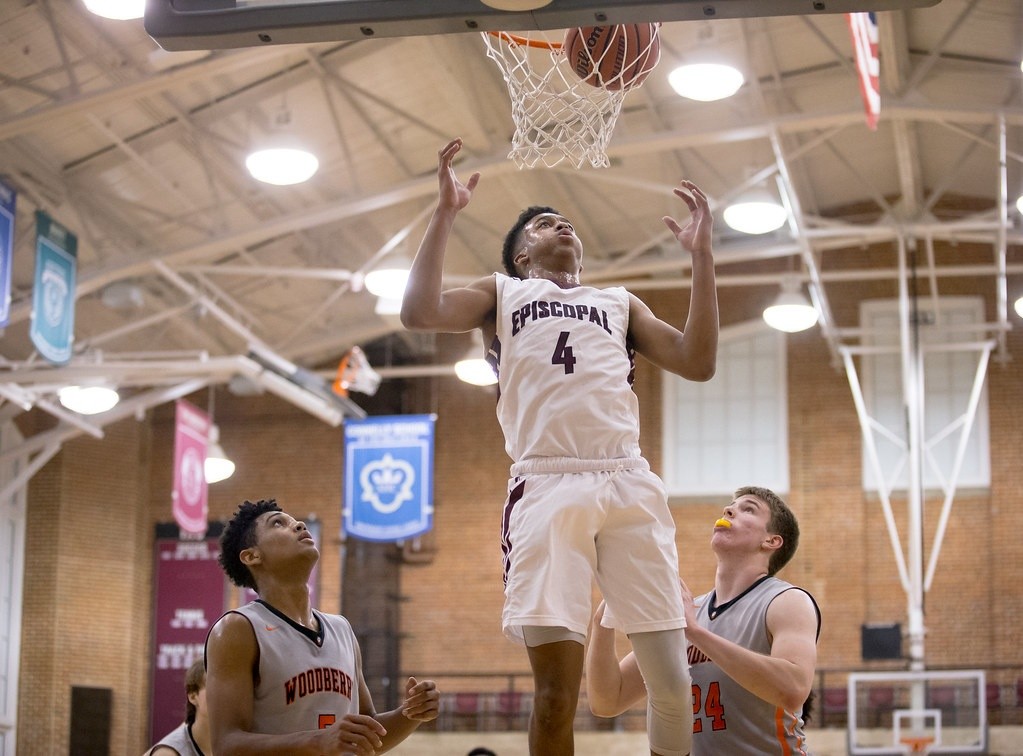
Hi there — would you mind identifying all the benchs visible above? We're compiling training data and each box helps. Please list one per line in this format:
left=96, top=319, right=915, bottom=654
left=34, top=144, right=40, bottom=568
left=427, top=682, right=1022, bottom=730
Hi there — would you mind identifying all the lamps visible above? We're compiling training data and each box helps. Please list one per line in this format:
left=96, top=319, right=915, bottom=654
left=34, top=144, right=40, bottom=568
left=666, top=23, right=819, bottom=333
left=62, top=51, right=499, bottom=482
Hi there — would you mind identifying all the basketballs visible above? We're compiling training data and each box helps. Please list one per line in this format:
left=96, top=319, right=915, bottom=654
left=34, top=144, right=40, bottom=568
left=565, top=22, right=660, bottom=90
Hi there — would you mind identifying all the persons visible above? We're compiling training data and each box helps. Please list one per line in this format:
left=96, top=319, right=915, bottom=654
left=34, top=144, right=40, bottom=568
left=148, top=657, right=213, bottom=756
left=399, top=136, right=721, bottom=756
left=586, top=487, right=821, bottom=756
left=468, top=748, right=496, bottom=756
left=203, top=498, right=440, bottom=756
